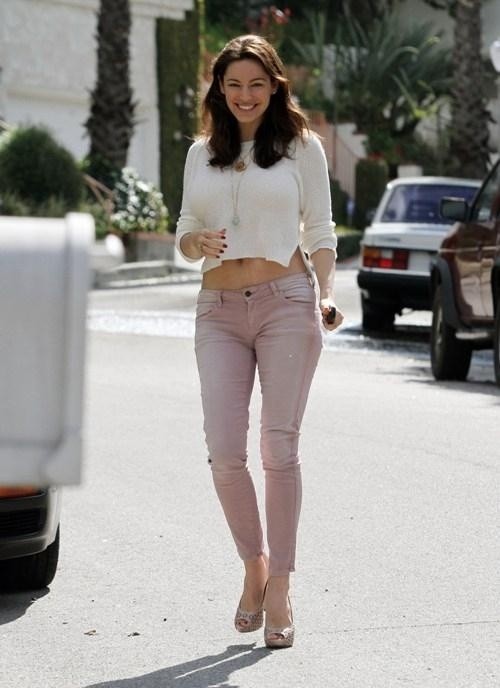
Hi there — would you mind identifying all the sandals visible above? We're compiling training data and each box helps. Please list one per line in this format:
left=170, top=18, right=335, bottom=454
left=263, top=584, right=295, bottom=648
left=235, top=550, right=270, bottom=633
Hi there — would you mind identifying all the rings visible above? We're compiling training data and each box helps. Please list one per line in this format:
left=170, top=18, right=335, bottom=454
left=198, top=244, right=206, bottom=253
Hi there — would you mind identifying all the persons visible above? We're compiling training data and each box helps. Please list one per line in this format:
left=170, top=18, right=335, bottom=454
left=172, top=35, right=345, bottom=649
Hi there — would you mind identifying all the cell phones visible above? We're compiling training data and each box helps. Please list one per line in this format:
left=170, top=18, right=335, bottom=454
left=325, top=307, right=335, bottom=324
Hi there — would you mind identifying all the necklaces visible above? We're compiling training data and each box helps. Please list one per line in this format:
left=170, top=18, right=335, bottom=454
left=227, top=138, right=255, bottom=173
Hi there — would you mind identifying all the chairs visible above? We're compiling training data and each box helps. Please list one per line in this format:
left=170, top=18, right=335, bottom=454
left=401, top=199, right=440, bottom=224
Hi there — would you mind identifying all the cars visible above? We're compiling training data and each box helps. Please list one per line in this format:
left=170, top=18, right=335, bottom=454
left=357, top=175, right=499, bottom=343
left=426, top=156, right=499, bottom=390
